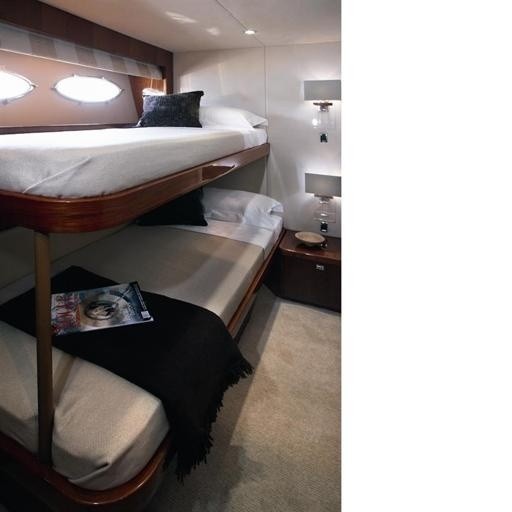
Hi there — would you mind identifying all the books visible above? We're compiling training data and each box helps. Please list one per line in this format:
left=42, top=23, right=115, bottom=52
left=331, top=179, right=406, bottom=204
left=49, top=281, right=154, bottom=336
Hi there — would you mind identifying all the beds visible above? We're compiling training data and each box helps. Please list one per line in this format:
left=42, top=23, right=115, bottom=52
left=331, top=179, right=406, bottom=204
left=0, top=129, right=286, bottom=512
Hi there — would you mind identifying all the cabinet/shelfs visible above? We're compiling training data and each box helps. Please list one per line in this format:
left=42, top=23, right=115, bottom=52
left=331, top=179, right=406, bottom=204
left=274, top=229, right=341, bottom=313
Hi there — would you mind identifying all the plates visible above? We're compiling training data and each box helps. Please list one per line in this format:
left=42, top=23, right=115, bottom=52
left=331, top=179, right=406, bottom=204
left=295, top=231, right=324, bottom=246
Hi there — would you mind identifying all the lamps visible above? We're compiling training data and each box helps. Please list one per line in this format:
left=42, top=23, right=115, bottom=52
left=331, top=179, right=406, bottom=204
left=303, top=80, right=341, bottom=143
left=305, top=173, right=341, bottom=234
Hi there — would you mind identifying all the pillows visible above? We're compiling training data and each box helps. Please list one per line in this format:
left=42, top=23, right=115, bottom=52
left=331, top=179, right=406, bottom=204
left=198, top=107, right=266, bottom=128
left=136, top=186, right=208, bottom=226
left=202, top=187, right=283, bottom=226
left=136, top=90, right=204, bottom=127
